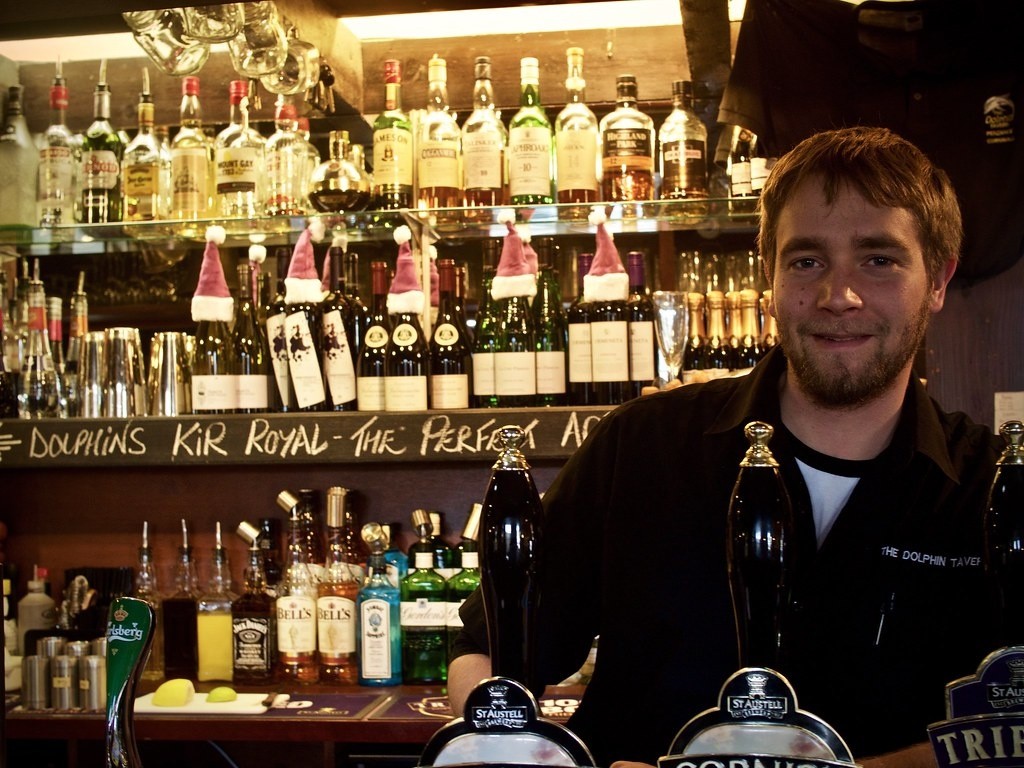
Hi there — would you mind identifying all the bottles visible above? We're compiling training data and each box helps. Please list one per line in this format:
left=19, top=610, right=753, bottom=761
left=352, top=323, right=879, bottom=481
left=985, top=418, right=1023, bottom=643
left=0, top=47, right=784, bottom=714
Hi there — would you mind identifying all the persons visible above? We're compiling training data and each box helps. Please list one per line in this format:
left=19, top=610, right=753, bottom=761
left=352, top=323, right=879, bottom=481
left=447, top=126, right=1024, bottom=768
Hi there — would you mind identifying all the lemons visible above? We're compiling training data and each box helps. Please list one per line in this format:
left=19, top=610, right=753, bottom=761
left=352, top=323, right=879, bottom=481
left=152, top=678, right=237, bottom=707
